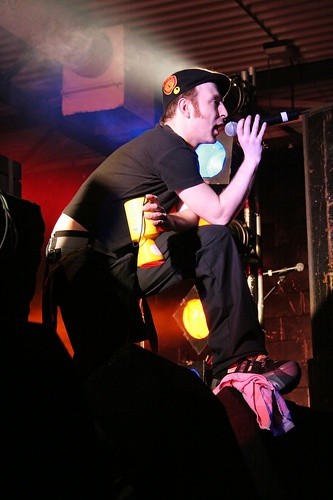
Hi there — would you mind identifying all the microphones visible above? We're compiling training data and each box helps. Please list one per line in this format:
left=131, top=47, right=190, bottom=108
left=263, top=262, right=304, bottom=276
left=224, top=110, right=310, bottom=137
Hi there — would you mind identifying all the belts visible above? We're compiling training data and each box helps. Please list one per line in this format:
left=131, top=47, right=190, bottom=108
left=46, top=234, right=118, bottom=258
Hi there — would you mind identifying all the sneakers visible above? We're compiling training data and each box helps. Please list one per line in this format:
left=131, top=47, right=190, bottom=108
left=210, top=358, right=302, bottom=394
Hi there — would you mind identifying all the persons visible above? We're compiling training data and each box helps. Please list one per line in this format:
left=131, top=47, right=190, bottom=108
left=44, top=68, right=304, bottom=403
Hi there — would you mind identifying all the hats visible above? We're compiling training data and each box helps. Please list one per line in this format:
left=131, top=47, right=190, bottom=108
left=161, top=68, right=231, bottom=112
